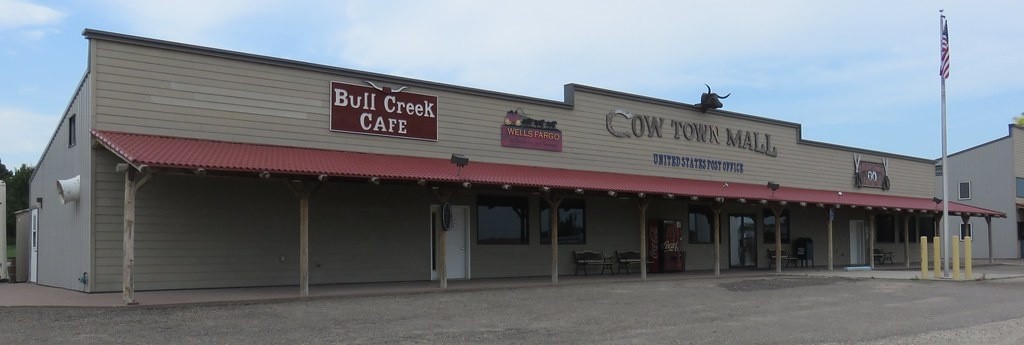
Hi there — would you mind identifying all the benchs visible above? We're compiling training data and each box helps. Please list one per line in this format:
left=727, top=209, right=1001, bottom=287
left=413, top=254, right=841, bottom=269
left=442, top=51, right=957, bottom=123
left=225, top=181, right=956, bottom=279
left=768, top=248, right=798, bottom=269
left=573, top=250, right=615, bottom=275
left=617, top=250, right=654, bottom=274
left=873, top=248, right=893, bottom=264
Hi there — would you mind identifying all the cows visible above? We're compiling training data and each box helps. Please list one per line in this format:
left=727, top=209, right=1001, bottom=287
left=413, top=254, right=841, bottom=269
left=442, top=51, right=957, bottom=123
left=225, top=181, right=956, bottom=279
left=700, top=83, right=732, bottom=109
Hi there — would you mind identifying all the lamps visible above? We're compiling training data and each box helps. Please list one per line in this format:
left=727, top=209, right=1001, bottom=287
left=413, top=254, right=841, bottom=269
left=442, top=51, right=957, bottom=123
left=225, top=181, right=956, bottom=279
left=767, top=179, right=779, bottom=191
left=932, top=196, right=943, bottom=208
left=451, top=154, right=469, bottom=174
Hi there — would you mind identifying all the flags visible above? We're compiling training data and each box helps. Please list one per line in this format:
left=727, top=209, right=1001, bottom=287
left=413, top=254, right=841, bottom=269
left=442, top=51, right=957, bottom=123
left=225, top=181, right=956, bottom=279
left=939, top=15, right=950, bottom=80
